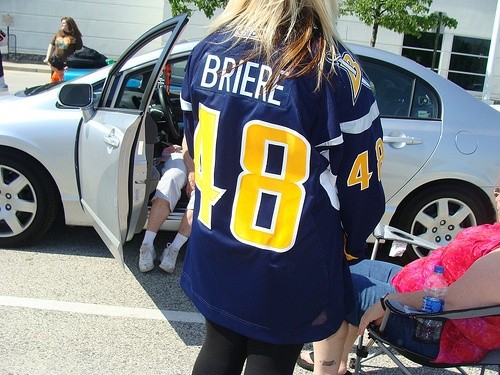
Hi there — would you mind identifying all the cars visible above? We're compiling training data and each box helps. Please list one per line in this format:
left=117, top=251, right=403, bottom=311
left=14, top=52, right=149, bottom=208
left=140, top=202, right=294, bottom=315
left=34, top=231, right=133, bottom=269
left=0, top=12, right=500, bottom=270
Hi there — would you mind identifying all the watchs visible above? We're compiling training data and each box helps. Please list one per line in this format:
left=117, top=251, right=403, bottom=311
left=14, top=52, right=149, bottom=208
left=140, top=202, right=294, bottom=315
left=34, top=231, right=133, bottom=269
left=380, top=293, right=388, bottom=310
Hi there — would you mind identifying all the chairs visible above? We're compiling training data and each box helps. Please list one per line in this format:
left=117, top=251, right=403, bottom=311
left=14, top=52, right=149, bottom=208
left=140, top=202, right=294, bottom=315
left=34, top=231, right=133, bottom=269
left=352, top=223, right=500, bottom=375
left=374, top=82, right=436, bottom=121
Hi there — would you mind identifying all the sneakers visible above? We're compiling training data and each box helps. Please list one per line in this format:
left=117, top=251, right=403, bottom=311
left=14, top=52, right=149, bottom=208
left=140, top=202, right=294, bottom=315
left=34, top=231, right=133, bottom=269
left=139, top=244, right=156, bottom=272
left=159, top=243, right=178, bottom=273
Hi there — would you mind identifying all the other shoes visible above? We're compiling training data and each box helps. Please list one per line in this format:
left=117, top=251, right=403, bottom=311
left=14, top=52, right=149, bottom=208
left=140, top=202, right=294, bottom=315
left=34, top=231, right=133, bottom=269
left=297, top=349, right=314, bottom=372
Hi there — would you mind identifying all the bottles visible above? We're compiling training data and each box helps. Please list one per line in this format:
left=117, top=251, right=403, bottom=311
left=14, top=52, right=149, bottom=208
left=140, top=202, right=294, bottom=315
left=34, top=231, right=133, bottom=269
left=416, top=266, right=448, bottom=341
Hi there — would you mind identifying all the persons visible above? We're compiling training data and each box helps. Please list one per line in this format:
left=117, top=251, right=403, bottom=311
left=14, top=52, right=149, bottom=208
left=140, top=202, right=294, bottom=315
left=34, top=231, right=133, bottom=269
left=139, top=129, right=195, bottom=273
left=297, top=188, right=500, bottom=375
left=180, top=0, right=386, bottom=375
left=44, top=17, right=83, bottom=83
